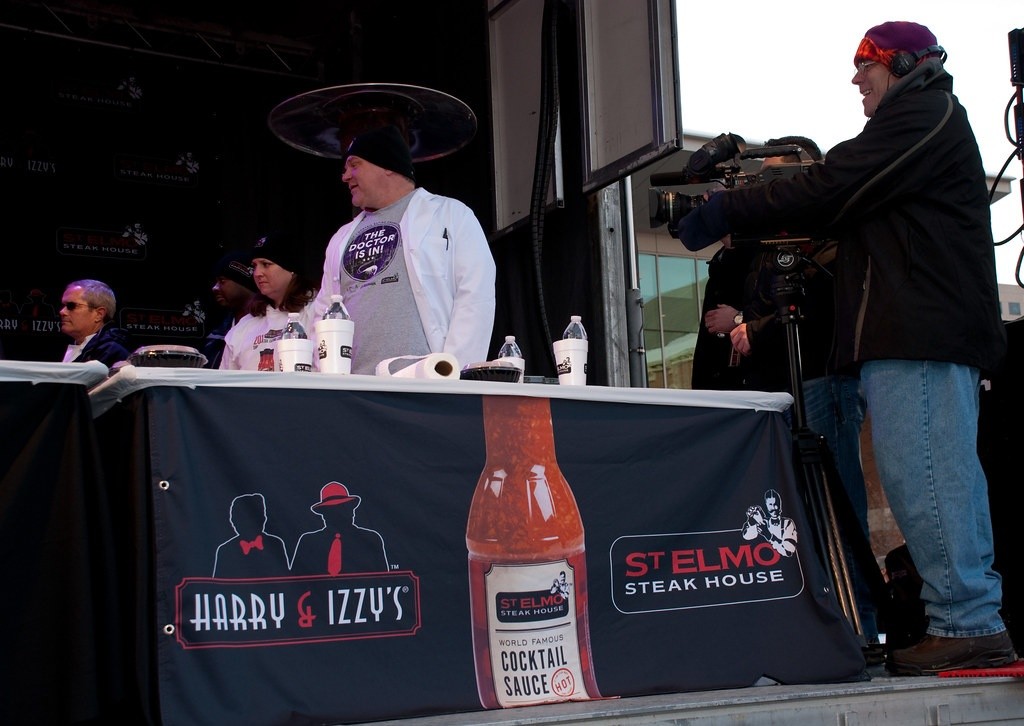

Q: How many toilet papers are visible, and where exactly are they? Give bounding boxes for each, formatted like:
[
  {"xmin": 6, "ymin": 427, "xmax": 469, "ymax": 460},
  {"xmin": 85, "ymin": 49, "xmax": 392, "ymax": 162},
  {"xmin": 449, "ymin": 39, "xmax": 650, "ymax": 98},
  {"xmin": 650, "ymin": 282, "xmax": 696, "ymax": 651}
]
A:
[{"xmin": 374, "ymin": 353, "xmax": 459, "ymax": 382}]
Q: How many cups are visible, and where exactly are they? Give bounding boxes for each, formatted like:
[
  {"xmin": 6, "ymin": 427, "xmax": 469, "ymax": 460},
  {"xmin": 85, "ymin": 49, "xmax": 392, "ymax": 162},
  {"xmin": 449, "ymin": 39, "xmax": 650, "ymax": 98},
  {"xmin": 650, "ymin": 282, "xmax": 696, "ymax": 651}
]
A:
[
  {"xmin": 314, "ymin": 319, "xmax": 356, "ymax": 374},
  {"xmin": 552, "ymin": 338, "xmax": 587, "ymax": 384},
  {"xmin": 277, "ymin": 338, "xmax": 313, "ymax": 372},
  {"xmin": 499, "ymin": 356, "xmax": 525, "ymax": 384}
]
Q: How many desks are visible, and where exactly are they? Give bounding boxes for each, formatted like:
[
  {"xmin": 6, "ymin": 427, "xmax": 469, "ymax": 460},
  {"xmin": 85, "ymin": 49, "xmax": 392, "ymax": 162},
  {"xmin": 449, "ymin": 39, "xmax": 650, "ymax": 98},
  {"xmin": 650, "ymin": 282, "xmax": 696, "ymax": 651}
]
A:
[
  {"xmin": 91, "ymin": 363, "xmax": 866, "ymax": 726},
  {"xmin": 0, "ymin": 359, "xmax": 134, "ymax": 726}
]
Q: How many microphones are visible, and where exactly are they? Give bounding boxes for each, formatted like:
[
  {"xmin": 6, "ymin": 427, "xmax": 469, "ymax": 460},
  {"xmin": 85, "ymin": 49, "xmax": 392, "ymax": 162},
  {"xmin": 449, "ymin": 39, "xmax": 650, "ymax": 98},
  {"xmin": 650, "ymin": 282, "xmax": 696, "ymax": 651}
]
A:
[{"xmin": 650, "ymin": 167, "xmax": 726, "ymax": 186}]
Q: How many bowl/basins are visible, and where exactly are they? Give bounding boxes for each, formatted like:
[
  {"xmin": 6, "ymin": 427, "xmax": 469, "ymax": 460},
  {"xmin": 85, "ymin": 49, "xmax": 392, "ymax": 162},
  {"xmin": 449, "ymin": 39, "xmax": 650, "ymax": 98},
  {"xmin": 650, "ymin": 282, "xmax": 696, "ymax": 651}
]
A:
[{"xmin": 128, "ymin": 345, "xmax": 208, "ymax": 369}]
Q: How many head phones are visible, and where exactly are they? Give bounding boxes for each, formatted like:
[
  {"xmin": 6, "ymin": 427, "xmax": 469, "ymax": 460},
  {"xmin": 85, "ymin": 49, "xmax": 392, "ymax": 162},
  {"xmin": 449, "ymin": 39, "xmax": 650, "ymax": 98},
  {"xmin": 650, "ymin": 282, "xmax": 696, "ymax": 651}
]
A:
[{"xmin": 888, "ymin": 45, "xmax": 948, "ymax": 78}]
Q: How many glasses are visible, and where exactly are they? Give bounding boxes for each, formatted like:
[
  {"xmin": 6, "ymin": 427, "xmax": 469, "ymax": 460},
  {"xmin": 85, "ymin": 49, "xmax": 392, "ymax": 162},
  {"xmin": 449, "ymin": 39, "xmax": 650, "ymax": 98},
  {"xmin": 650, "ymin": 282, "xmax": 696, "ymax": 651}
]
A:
[
  {"xmin": 854, "ymin": 62, "xmax": 878, "ymax": 75},
  {"xmin": 58, "ymin": 302, "xmax": 97, "ymax": 310}
]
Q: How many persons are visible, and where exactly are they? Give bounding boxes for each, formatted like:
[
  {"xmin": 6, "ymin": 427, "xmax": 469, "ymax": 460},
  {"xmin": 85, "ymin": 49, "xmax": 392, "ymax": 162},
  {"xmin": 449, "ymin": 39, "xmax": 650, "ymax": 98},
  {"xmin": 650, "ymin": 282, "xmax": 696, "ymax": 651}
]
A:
[
  {"xmin": 203, "ymin": 240, "xmax": 319, "ymax": 371},
  {"xmin": 691, "ymin": 136, "xmax": 885, "ymax": 666},
  {"xmin": 59, "ymin": 279, "xmax": 133, "ymax": 368},
  {"xmin": 678, "ymin": 21, "xmax": 1018, "ymax": 676},
  {"xmin": 313, "ymin": 126, "xmax": 496, "ymax": 376}
]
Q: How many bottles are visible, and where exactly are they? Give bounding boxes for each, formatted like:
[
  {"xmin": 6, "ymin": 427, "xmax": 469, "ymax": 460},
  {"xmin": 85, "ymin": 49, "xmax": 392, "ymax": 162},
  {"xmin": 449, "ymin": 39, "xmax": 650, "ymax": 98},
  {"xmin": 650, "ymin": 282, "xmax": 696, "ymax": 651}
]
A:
[
  {"xmin": 562, "ymin": 315, "xmax": 587, "ymax": 340},
  {"xmin": 282, "ymin": 312, "xmax": 308, "ymax": 339},
  {"xmin": 322, "ymin": 295, "xmax": 352, "ymax": 320},
  {"xmin": 498, "ymin": 335, "xmax": 522, "ymax": 359},
  {"xmin": 466, "ymin": 395, "xmax": 602, "ymax": 709}
]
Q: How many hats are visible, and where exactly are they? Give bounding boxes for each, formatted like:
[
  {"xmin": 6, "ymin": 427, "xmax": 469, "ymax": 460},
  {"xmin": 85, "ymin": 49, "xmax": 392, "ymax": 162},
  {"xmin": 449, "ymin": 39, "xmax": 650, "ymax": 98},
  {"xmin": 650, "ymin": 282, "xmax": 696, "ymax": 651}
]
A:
[
  {"xmin": 213, "ymin": 253, "xmax": 257, "ymax": 293},
  {"xmin": 854, "ymin": 21, "xmax": 939, "ymax": 80},
  {"xmin": 342, "ymin": 126, "xmax": 416, "ymax": 182},
  {"xmin": 250, "ymin": 232, "xmax": 310, "ymax": 276}
]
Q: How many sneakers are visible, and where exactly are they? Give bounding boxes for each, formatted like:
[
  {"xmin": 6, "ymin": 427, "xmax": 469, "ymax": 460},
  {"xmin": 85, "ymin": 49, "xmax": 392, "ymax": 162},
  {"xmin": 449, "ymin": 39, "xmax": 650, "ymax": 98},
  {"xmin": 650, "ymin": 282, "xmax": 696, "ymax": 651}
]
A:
[{"xmin": 886, "ymin": 629, "xmax": 1019, "ymax": 676}]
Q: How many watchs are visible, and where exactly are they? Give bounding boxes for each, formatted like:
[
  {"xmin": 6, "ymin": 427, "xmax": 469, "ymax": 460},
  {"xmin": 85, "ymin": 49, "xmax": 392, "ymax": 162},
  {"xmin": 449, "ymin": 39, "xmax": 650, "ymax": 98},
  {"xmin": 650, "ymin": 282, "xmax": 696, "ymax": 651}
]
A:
[{"xmin": 733, "ymin": 311, "xmax": 743, "ymax": 326}]
